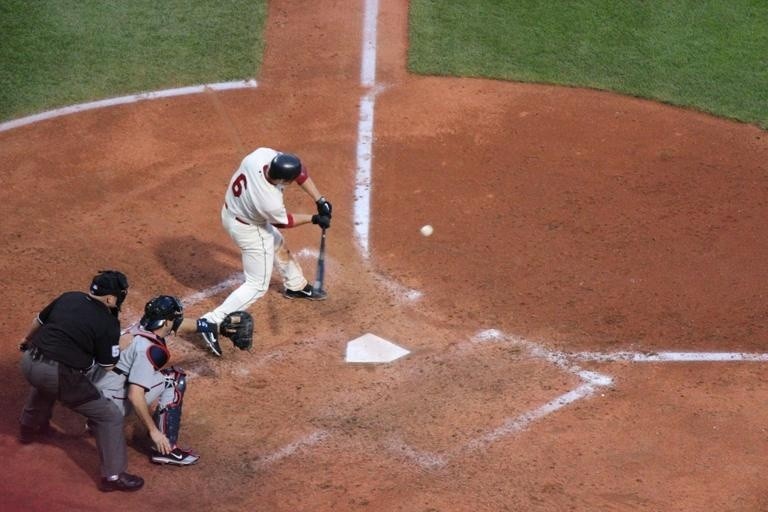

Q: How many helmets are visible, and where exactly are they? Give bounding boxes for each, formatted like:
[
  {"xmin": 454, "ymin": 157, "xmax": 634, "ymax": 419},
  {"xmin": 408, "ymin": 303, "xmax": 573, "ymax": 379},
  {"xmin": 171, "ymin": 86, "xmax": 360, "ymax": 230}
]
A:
[
  {"xmin": 270, "ymin": 153, "xmax": 302, "ymax": 182},
  {"xmin": 89, "ymin": 268, "xmax": 129, "ymax": 312},
  {"xmin": 138, "ymin": 296, "xmax": 184, "ymax": 337}
]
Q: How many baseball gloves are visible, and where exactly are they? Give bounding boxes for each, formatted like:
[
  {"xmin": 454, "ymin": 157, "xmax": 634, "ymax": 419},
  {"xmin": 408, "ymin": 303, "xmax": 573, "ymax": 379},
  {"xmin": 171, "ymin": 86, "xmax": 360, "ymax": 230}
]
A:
[{"xmin": 220, "ymin": 311, "xmax": 254, "ymax": 349}]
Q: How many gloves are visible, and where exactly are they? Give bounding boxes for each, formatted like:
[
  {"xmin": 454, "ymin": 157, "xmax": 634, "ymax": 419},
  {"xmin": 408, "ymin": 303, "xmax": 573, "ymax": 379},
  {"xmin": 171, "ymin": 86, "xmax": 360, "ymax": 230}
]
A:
[{"xmin": 311, "ymin": 196, "xmax": 333, "ymax": 229}]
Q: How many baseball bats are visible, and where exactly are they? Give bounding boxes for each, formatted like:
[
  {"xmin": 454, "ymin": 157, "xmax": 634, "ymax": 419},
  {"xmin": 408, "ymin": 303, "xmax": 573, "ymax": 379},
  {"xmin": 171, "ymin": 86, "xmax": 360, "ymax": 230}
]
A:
[{"xmin": 312, "ymin": 227, "xmax": 326, "ymax": 296}]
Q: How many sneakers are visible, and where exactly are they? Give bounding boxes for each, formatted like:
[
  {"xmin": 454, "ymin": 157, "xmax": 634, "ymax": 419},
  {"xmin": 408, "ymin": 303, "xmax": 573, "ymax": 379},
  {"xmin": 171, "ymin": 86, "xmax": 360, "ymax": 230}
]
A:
[
  {"xmin": 195, "ymin": 316, "xmax": 224, "ymax": 359},
  {"xmin": 283, "ymin": 283, "xmax": 328, "ymax": 302},
  {"xmin": 99, "ymin": 472, "xmax": 146, "ymax": 492},
  {"xmin": 15, "ymin": 422, "xmax": 53, "ymax": 443},
  {"xmin": 149, "ymin": 441, "xmax": 199, "ymax": 467}
]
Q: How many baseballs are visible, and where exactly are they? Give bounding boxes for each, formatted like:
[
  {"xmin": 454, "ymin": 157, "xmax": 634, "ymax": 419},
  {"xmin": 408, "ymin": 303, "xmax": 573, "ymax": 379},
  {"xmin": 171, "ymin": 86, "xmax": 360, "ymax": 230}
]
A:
[{"xmin": 420, "ymin": 225, "xmax": 433, "ymax": 236}]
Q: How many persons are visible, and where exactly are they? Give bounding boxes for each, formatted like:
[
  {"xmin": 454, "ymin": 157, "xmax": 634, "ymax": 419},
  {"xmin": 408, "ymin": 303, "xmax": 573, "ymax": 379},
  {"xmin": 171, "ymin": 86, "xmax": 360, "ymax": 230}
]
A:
[
  {"xmin": 83, "ymin": 294, "xmax": 213, "ymax": 466},
  {"xmin": 17, "ymin": 270, "xmax": 144, "ymax": 492},
  {"xmin": 199, "ymin": 147, "xmax": 332, "ymax": 357}
]
